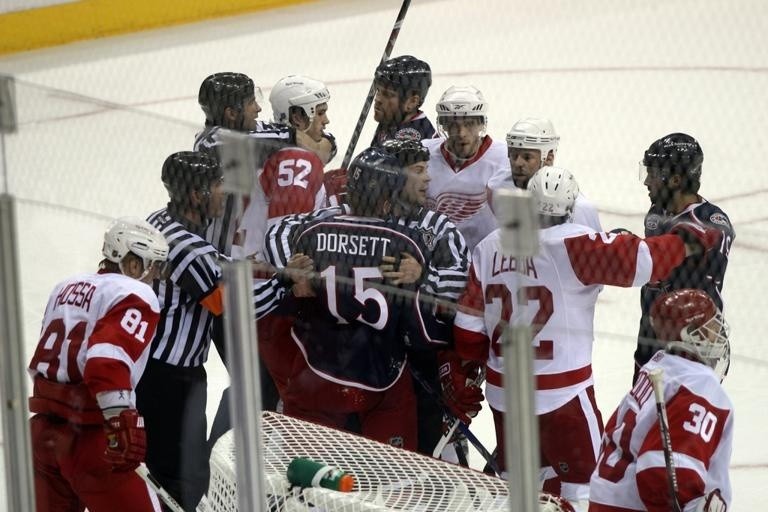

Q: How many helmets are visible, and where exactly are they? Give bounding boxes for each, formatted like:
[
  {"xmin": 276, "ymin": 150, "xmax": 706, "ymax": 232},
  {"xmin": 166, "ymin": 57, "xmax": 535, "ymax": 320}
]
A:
[
  {"xmin": 346, "ymin": 147, "xmax": 408, "ymax": 216},
  {"xmin": 505, "ymin": 118, "xmax": 560, "ymax": 162},
  {"xmin": 101, "ymin": 216, "xmax": 170, "ymax": 265},
  {"xmin": 638, "ymin": 132, "xmax": 704, "ymax": 181},
  {"xmin": 162, "ymin": 151, "xmax": 224, "ymax": 214},
  {"xmin": 269, "ymin": 73, "xmax": 331, "ymax": 123},
  {"xmin": 526, "ymin": 165, "xmax": 579, "ymax": 218},
  {"xmin": 436, "ymin": 84, "xmax": 489, "ymax": 138},
  {"xmin": 374, "ymin": 54, "xmax": 432, "ymax": 93},
  {"xmin": 198, "ymin": 71, "xmax": 254, "ymax": 123},
  {"xmin": 649, "ymin": 288, "xmax": 731, "ymax": 365},
  {"xmin": 379, "ymin": 126, "xmax": 431, "ymax": 167}
]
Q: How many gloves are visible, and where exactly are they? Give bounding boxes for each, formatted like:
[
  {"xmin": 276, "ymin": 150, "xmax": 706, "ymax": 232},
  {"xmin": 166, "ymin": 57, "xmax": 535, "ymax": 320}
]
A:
[
  {"xmin": 670, "ymin": 220, "xmax": 722, "ymax": 256},
  {"xmin": 103, "ymin": 408, "xmax": 147, "ymax": 474},
  {"xmin": 436, "ymin": 348, "xmax": 485, "ymax": 425},
  {"xmin": 324, "ymin": 168, "xmax": 351, "ymax": 205}
]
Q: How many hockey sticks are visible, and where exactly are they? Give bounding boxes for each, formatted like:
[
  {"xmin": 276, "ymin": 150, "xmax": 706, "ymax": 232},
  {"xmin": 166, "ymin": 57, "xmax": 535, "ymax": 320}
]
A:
[
  {"xmin": 379, "ymin": 364, "xmax": 488, "ymax": 492},
  {"xmin": 404, "ymin": 357, "xmax": 562, "ymax": 481}
]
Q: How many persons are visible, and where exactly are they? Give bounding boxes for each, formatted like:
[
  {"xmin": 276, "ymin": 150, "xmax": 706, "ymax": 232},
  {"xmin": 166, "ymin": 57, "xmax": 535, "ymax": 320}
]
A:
[
  {"xmin": 630, "ymin": 131, "xmax": 739, "ymax": 388},
  {"xmin": 588, "ymin": 285, "xmax": 734, "ymax": 511},
  {"xmin": 134, "ymin": 149, "xmax": 316, "ymax": 512},
  {"xmin": 452, "ymin": 161, "xmax": 727, "ymax": 511},
  {"xmin": 189, "ymin": 55, "xmax": 601, "ymax": 511},
  {"xmin": 27, "ymin": 213, "xmax": 174, "ymax": 511}
]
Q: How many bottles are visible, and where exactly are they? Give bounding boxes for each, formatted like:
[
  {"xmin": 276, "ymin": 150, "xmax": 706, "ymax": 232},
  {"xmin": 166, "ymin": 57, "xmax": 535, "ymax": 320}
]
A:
[{"xmin": 284, "ymin": 458, "xmax": 354, "ymax": 492}]
